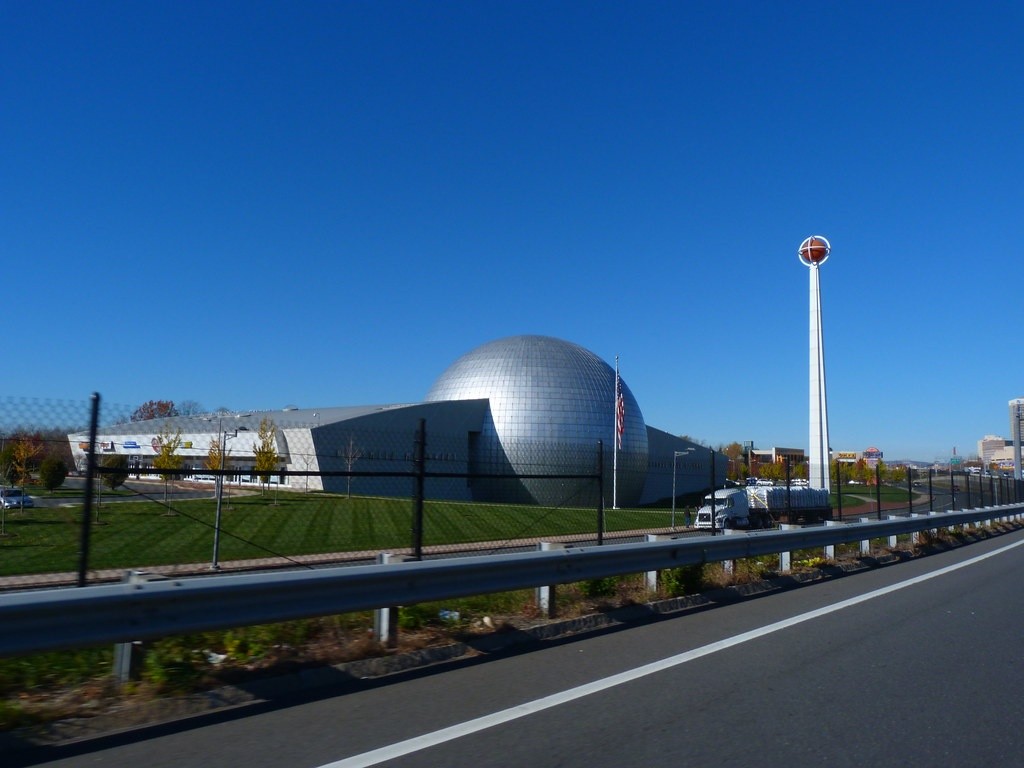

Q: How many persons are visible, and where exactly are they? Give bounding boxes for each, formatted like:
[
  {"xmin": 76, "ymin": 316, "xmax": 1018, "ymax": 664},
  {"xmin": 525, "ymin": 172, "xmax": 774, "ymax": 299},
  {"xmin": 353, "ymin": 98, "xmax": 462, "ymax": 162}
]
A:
[
  {"xmin": 695, "ymin": 505, "xmax": 703, "ymax": 515},
  {"xmin": 683, "ymin": 504, "xmax": 692, "ymax": 528}
]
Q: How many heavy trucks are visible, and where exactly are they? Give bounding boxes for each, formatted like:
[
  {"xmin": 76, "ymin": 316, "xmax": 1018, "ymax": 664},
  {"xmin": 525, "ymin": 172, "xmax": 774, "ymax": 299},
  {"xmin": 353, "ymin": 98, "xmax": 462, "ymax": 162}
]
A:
[{"xmin": 694, "ymin": 485, "xmax": 834, "ymax": 530}]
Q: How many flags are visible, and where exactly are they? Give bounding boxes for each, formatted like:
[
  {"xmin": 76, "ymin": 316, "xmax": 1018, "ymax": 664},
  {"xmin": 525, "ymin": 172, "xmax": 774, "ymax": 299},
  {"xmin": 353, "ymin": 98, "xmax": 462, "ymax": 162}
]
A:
[{"xmin": 615, "ymin": 366, "xmax": 625, "ymax": 449}]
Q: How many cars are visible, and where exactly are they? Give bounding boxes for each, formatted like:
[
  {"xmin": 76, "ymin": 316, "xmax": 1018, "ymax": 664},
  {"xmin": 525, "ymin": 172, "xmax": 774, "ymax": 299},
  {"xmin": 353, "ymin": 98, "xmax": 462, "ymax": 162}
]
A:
[
  {"xmin": 950, "ymin": 485, "xmax": 960, "ymax": 494},
  {"xmin": 848, "ymin": 480, "xmax": 863, "ymax": 485},
  {"xmin": 973, "ymin": 469, "xmax": 1011, "ymax": 477},
  {"xmin": 914, "ymin": 482, "xmax": 922, "ymax": 487},
  {"xmin": 725, "ymin": 477, "xmax": 810, "ymax": 486},
  {"xmin": 0, "ymin": 488, "xmax": 34, "ymax": 510}
]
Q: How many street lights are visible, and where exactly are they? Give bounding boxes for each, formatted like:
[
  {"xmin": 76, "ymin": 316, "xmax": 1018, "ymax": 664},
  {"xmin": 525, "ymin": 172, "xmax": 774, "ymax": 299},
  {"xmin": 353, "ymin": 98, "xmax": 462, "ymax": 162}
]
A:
[
  {"xmin": 210, "ymin": 426, "xmax": 249, "ymax": 571},
  {"xmin": 672, "ymin": 451, "xmax": 690, "ymax": 528}
]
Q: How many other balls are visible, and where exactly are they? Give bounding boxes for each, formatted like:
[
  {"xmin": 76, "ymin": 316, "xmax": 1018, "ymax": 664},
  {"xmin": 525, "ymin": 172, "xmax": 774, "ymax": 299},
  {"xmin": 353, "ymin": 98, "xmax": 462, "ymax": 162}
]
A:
[{"xmin": 804, "ymin": 240, "xmax": 826, "ymax": 261}]
